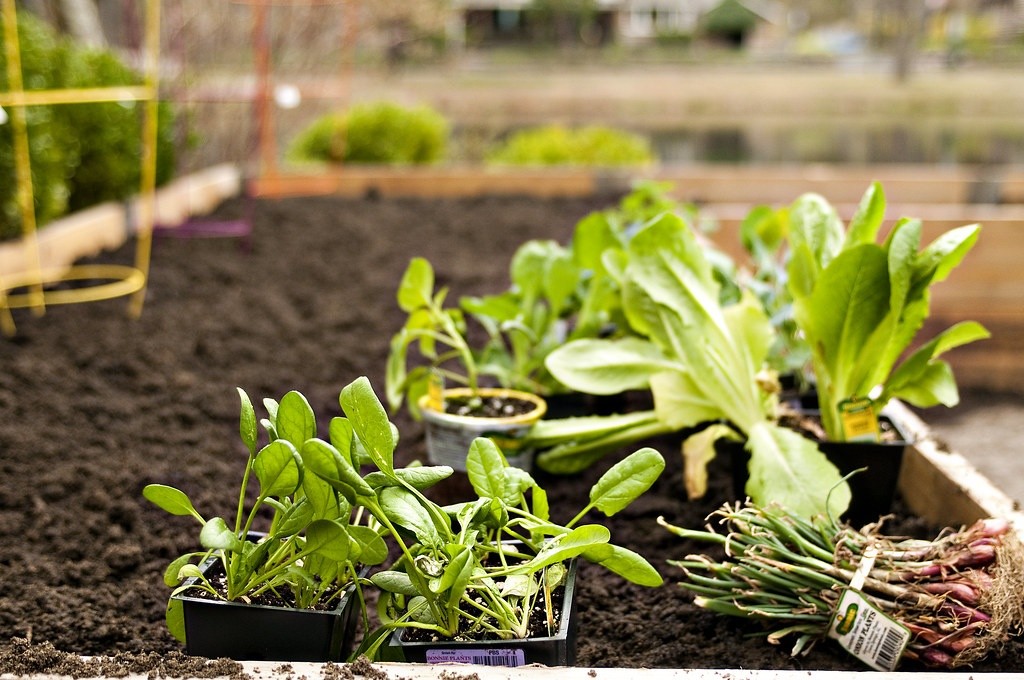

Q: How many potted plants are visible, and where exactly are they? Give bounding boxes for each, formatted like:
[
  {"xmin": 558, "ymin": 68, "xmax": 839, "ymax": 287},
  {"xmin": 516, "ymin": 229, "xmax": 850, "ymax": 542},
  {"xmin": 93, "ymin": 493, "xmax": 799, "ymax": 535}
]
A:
[
  {"xmin": 468, "ymin": 180, "xmax": 994, "ymax": 537},
  {"xmin": 382, "ymin": 256, "xmax": 546, "ymax": 478},
  {"xmin": 297, "ymin": 377, "xmax": 668, "ymax": 674},
  {"xmin": 145, "ymin": 383, "xmax": 456, "ymax": 667}
]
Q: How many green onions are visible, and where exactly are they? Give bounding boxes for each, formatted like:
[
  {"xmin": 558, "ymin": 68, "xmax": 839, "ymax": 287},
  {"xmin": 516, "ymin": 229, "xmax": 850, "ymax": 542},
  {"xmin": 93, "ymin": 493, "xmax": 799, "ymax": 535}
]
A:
[{"xmin": 646, "ymin": 464, "xmax": 1022, "ymax": 672}]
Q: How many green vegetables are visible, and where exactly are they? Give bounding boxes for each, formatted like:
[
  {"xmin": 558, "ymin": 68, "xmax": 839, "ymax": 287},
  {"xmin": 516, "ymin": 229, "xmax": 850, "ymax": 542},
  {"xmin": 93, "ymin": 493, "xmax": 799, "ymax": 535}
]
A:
[
  {"xmin": 120, "ymin": 375, "xmax": 668, "ymax": 634},
  {"xmin": 383, "ymin": 170, "xmax": 988, "ymax": 507}
]
